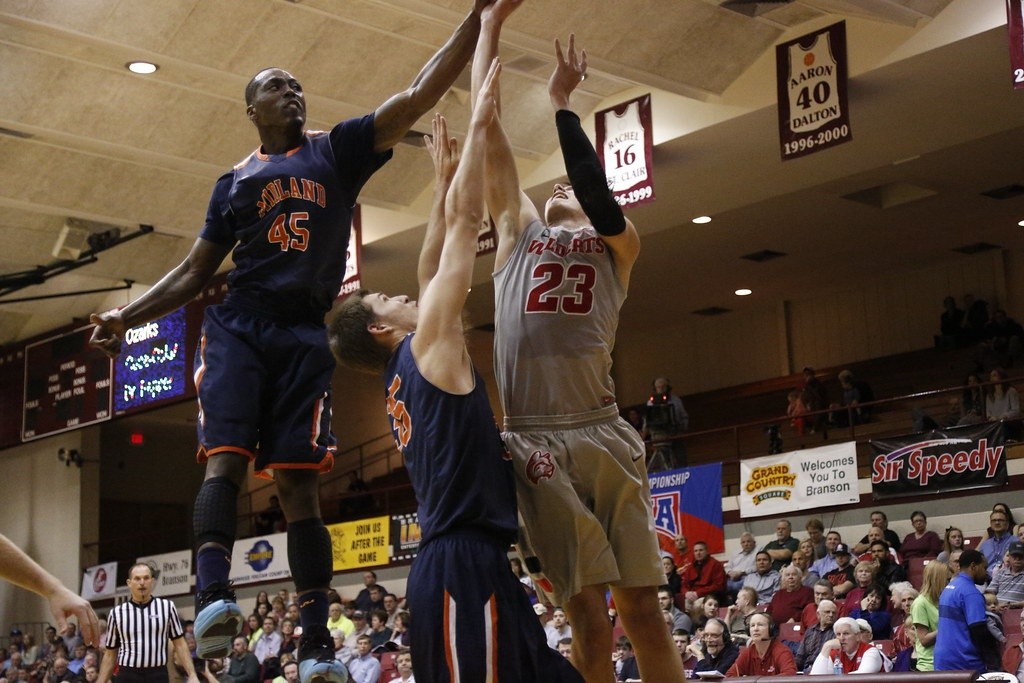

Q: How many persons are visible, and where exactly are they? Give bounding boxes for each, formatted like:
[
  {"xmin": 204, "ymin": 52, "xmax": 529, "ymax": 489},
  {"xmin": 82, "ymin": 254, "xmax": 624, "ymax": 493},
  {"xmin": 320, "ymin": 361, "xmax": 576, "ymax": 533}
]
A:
[
  {"xmin": 725, "ymin": 611, "xmax": 796, "ymax": 678},
  {"xmin": 95, "ymin": 562, "xmax": 199, "ymax": 683},
  {"xmin": 0, "ymin": 294, "xmax": 1024, "ymax": 683},
  {"xmin": 933, "ymin": 549, "xmax": 1005, "ymax": 678},
  {"xmin": 0, "ymin": 533, "xmax": 100, "ymax": 650},
  {"xmin": 809, "ymin": 617, "xmax": 888, "ymax": 675},
  {"xmin": 470, "ymin": 0, "xmax": 686, "ymax": 683},
  {"xmin": 87, "ymin": 0, "xmax": 500, "ymax": 683},
  {"xmin": 327, "ymin": 54, "xmax": 586, "ymax": 683}
]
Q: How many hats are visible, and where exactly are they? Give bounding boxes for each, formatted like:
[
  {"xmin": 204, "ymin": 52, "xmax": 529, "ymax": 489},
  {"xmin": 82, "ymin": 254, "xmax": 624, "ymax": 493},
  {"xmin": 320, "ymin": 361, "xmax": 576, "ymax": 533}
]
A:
[
  {"xmin": 519, "ymin": 577, "xmax": 532, "ymax": 590},
  {"xmin": 353, "ymin": 609, "xmax": 367, "ymax": 618},
  {"xmin": 832, "ymin": 543, "xmax": 852, "ymax": 555},
  {"xmin": 856, "ymin": 619, "xmax": 872, "ymax": 633},
  {"xmin": 1009, "ymin": 541, "xmax": 1024, "ymax": 555},
  {"xmin": 10, "ymin": 629, "xmax": 23, "ymax": 638},
  {"xmin": 292, "ymin": 627, "xmax": 302, "ymax": 637},
  {"xmin": 533, "ymin": 604, "xmax": 547, "ymax": 615}
]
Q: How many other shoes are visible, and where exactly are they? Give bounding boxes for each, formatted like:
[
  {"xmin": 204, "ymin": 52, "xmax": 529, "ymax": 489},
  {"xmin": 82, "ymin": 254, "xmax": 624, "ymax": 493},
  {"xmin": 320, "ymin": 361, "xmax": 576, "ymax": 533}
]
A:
[{"xmin": 608, "ymin": 615, "xmax": 616, "ymax": 627}]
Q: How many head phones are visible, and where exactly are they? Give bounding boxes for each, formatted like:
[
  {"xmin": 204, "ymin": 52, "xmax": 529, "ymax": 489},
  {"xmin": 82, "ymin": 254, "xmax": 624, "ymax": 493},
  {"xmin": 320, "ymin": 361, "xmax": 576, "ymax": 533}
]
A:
[
  {"xmin": 713, "ymin": 617, "xmax": 730, "ymax": 644},
  {"xmin": 744, "ymin": 612, "xmax": 780, "ymax": 637}
]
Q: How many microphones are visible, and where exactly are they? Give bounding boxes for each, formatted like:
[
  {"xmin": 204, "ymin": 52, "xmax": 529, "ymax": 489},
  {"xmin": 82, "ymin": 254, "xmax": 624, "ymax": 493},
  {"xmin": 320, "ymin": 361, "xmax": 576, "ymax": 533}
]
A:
[{"xmin": 761, "ymin": 632, "xmax": 779, "ymax": 641}]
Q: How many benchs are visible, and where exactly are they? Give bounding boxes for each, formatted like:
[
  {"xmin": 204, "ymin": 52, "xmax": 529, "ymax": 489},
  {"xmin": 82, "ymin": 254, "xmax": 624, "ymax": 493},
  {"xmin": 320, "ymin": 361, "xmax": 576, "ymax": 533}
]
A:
[
  {"xmin": 323, "ymin": 463, "xmax": 416, "ymax": 516},
  {"xmin": 618, "ymin": 335, "xmax": 1024, "ymax": 496}
]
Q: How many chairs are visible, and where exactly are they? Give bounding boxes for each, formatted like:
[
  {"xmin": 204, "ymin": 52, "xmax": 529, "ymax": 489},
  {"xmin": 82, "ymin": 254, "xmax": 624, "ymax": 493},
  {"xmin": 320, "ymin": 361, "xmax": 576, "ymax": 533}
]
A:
[
  {"xmin": 963, "ymin": 536, "xmax": 982, "ymax": 550},
  {"xmin": 379, "ymin": 651, "xmax": 400, "ymax": 682},
  {"xmin": 874, "ymin": 640, "xmax": 894, "ymax": 656},
  {"xmin": 908, "ymin": 557, "xmax": 937, "ymax": 593},
  {"xmin": 779, "ymin": 623, "xmax": 802, "ymax": 643}
]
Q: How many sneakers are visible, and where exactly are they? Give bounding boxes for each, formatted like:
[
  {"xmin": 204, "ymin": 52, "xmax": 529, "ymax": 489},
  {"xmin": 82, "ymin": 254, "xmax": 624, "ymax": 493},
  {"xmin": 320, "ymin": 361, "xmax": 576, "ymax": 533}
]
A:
[
  {"xmin": 297, "ymin": 624, "xmax": 348, "ymax": 683},
  {"xmin": 193, "ymin": 581, "xmax": 243, "ymax": 659}
]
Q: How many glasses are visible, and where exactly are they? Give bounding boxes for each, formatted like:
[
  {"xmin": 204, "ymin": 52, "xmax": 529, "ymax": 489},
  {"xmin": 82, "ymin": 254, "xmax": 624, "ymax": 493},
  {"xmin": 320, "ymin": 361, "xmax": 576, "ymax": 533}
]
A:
[
  {"xmin": 991, "ymin": 519, "xmax": 1008, "ymax": 523},
  {"xmin": 703, "ymin": 632, "xmax": 723, "ymax": 639}
]
